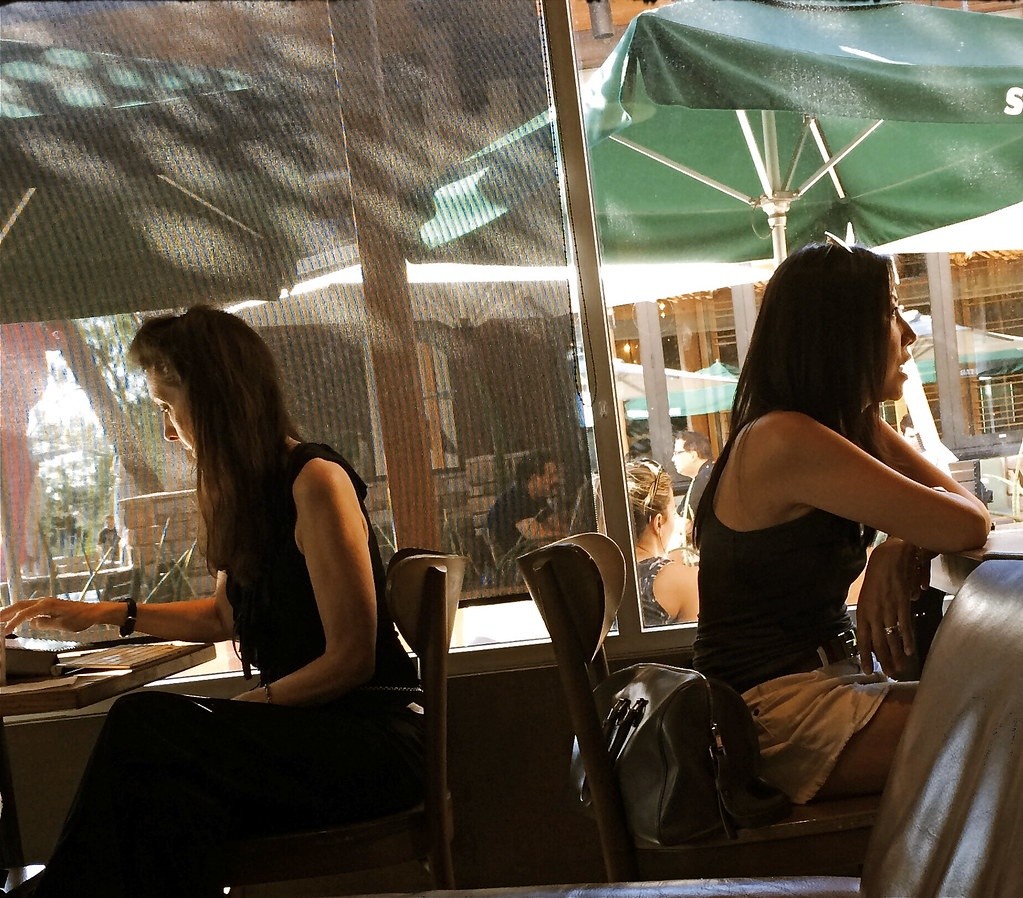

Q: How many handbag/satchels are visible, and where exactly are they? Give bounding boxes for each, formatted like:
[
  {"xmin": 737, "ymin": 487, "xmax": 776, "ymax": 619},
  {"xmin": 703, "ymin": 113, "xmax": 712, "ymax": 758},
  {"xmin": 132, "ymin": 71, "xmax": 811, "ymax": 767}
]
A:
[{"xmin": 570, "ymin": 659, "xmax": 791, "ymax": 847}]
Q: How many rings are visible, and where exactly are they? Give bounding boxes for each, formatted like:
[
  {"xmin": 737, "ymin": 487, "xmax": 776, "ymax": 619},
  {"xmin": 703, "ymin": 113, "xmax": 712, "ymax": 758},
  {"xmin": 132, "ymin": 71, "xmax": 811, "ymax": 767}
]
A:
[{"xmin": 884, "ymin": 625, "xmax": 898, "ymax": 635}]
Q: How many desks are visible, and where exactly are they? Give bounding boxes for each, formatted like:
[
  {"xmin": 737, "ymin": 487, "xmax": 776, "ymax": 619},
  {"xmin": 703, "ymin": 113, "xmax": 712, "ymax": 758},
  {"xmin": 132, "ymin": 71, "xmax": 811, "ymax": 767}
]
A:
[
  {"xmin": 919, "ymin": 523, "xmax": 1023, "ymax": 660},
  {"xmin": 2, "ymin": 642, "xmax": 218, "ymax": 896}
]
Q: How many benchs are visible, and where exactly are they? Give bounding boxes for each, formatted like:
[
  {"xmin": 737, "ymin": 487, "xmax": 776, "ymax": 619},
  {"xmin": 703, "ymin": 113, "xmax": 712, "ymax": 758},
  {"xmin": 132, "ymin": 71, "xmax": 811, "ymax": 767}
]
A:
[
  {"xmin": 949, "ymin": 460, "xmax": 982, "ymax": 497},
  {"xmin": 145, "ymin": 560, "xmax": 183, "ymax": 604},
  {"xmin": 222, "ymin": 545, "xmax": 468, "ymax": 896}
]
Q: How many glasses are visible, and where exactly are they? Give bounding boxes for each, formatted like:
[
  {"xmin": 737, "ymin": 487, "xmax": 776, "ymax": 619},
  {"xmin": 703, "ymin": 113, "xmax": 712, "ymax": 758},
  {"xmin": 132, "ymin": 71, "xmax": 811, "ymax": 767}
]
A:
[
  {"xmin": 672, "ymin": 451, "xmax": 689, "ymax": 456},
  {"xmin": 639, "ymin": 458, "xmax": 666, "ymax": 524},
  {"xmin": 823, "ymin": 230, "xmax": 853, "ymax": 259}
]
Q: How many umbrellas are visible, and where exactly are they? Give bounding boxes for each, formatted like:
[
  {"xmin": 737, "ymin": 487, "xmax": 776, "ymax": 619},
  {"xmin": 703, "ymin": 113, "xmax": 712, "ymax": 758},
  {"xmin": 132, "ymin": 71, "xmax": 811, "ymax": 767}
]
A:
[
  {"xmin": 616, "ymin": 360, "xmax": 745, "ymax": 457},
  {"xmin": 407, "ymin": 0, "xmax": 1023, "ymax": 276},
  {"xmin": 887, "ymin": 309, "xmax": 1023, "ymax": 393},
  {"xmin": 570, "ymin": 346, "xmax": 739, "ymax": 403},
  {"xmin": 0, "ymin": 41, "xmax": 311, "ymax": 327}
]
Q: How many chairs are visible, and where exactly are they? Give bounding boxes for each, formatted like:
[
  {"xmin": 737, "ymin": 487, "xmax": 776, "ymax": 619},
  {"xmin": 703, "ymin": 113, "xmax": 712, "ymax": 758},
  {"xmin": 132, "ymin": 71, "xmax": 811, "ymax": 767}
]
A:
[
  {"xmin": 517, "ymin": 531, "xmax": 879, "ymax": 881},
  {"xmin": 456, "ymin": 519, "xmax": 519, "ymax": 590},
  {"xmin": 101, "ymin": 567, "xmax": 141, "ymax": 604}
]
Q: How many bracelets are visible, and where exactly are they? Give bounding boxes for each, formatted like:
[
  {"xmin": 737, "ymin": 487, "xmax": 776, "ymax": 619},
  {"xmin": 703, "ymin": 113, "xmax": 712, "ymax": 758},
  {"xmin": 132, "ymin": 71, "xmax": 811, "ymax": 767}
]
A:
[
  {"xmin": 118, "ymin": 598, "xmax": 138, "ymax": 637},
  {"xmin": 261, "ymin": 683, "xmax": 276, "ymax": 706},
  {"xmin": 931, "ymin": 485, "xmax": 947, "ymax": 492}
]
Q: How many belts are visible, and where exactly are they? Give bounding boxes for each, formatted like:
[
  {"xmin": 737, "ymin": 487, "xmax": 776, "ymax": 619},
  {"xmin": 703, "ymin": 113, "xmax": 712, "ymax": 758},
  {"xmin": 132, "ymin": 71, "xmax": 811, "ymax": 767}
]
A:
[{"xmin": 737, "ymin": 624, "xmax": 861, "ymax": 694}]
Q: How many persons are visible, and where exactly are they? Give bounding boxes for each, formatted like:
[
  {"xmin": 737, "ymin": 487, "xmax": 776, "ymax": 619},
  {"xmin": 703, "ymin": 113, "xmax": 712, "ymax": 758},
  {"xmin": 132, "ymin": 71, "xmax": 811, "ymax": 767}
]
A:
[
  {"xmin": 672, "ymin": 430, "xmax": 718, "ymax": 517},
  {"xmin": 897, "ymin": 412, "xmax": 963, "ymax": 464},
  {"xmin": 572, "ymin": 458, "xmax": 702, "ymax": 634},
  {"xmin": 484, "ymin": 451, "xmax": 571, "ymax": 554},
  {"xmin": 0, "ymin": 301, "xmax": 405, "ymax": 897},
  {"xmin": 95, "ymin": 514, "xmax": 120, "ymax": 561},
  {"xmin": 684, "ymin": 237, "xmax": 996, "ymax": 805}
]
